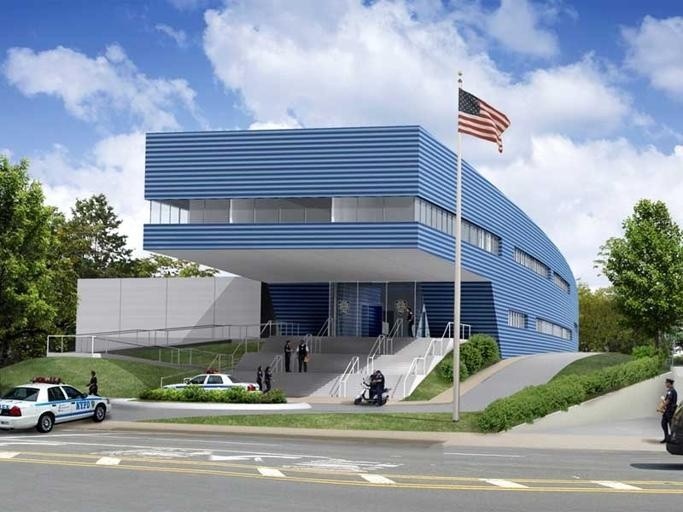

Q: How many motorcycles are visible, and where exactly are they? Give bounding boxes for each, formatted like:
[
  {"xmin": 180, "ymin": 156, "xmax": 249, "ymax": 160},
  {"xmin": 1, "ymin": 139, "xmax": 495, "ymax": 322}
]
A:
[{"xmin": 354, "ymin": 378, "xmax": 391, "ymax": 407}]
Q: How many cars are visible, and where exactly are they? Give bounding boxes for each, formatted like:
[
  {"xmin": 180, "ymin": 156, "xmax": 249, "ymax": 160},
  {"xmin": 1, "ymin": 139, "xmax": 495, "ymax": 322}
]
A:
[
  {"xmin": 163, "ymin": 369, "xmax": 259, "ymax": 393},
  {"xmin": 666, "ymin": 400, "xmax": 682, "ymax": 456},
  {"xmin": 0, "ymin": 376, "xmax": 111, "ymax": 434},
  {"xmin": 672, "ymin": 345, "xmax": 682, "ymax": 358}
]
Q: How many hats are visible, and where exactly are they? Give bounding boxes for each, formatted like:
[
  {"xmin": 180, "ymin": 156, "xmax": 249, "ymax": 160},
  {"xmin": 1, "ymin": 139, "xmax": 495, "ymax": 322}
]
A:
[{"xmin": 665, "ymin": 379, "xmax": 674, "ymax": 384}]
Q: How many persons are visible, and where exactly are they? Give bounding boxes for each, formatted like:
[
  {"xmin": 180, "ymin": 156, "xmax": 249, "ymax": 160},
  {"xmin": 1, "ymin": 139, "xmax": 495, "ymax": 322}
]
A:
[
  {"xmin": 85, "ymin": 370, "xmax": 100, "ymax": 396},
  {"xmin": 362, "ymin": 369, "xmax": 384, "ymax": 406},
  {"xmin": 256, "ymin": 365, "xmax": 264, "ymax": 391},
  {"xmin": 583, "ymin": 344, "xmax": 589, "ymax": 352},
  {"xmin": 660, "ymin": 378, "xmax": 678, "ymax": 444},
  {"xmin": 295, "ymin": 339, "xmax": 310, "ymax": 373},
  {"xmin": 283, "ymin": 339, "xmax": 294, "ymax": 373},
  {"xmin": 263, "ymin": 366, "xmax": 273, "ymax": 394},
  {"xmin": 406, "ymin": 306, "xmax": 414, "ymax": 337}
]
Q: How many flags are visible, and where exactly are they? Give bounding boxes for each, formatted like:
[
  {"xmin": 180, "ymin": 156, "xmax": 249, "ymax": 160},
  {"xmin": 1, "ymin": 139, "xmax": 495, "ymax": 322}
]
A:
[{"xmin": 457, "ymin": 86, "xmax": 511, "ymax": 154}]
